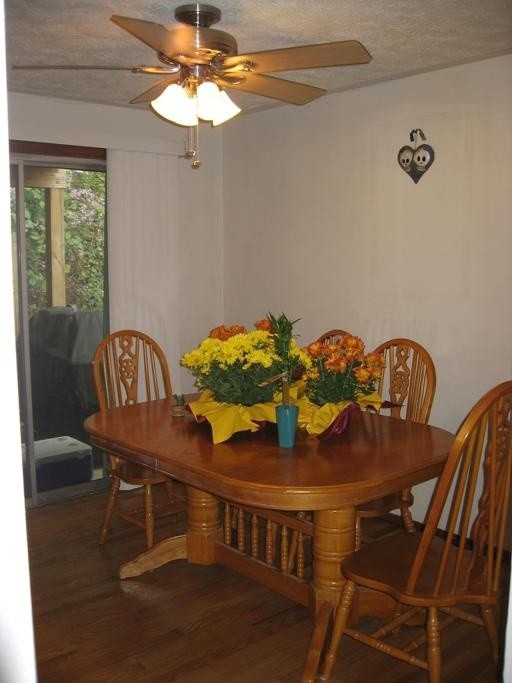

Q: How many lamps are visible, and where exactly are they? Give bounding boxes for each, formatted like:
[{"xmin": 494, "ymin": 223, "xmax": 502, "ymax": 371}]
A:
[{"xmin": 150, "ymin": 75, "xmax": 242, "ymax": 127}]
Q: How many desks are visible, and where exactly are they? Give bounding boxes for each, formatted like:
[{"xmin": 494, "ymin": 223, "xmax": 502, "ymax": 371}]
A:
[{"xmin": 82, "ymin": 394, "xmax": 457, "ymax": 683}]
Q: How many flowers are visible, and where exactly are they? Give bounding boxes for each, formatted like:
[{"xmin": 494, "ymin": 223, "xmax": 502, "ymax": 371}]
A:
[{"xmin": 181, "ymin": 310, "xmax": 389, "ymax": 405}]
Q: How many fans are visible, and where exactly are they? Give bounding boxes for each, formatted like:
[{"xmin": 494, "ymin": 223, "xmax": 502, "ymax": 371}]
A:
[{"xmin": 12, "ymin": 2, "xmax": 372, "ymax": 106}]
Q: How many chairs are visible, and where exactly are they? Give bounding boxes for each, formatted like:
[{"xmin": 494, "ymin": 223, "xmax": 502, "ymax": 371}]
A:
[
  {"xmin": 288, "ymin": 339, "xmax": 438, "ymax": 573},
  {"xmin": 319, "ymin": 381, "xmax": 511, "ymax": 681},
  {"xmin": 93, "ymin": 328, "xmax": 193, "ymax": 549},
  {"xmin": 224, "ymin": 334, "xmax": 362, "ymax": 557}
]
